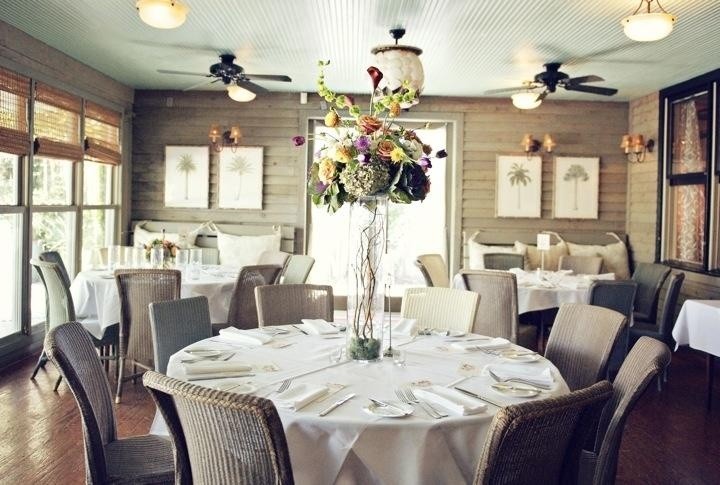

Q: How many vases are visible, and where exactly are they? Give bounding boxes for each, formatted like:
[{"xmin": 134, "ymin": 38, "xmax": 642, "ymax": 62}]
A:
[{"xmin": 345, "ymin": 195, "xmax": 387, "ymax": 358}]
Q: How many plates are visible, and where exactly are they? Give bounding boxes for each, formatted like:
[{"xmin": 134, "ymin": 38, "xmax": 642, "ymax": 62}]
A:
[
  {"xmin": 434, "ymin": 330, "xmax": 465, "ymax": 337},
  {"xmin": 184, "ymin": 348, "xmax": 222, "ymax": 358},
  {"xmin": 260, "ymin": 327, "xmax": 288, "ymax": 334},
  {"xmin": 368, "ymin": 399, "xmax": 415, "ymax": 417},
  {"xmin": 490, "ymin": 382, "xmax": 538, "ymax": 397},
  {"xmin": 500, "ymin": 351, "xmax": 540, "ymax": 363}
]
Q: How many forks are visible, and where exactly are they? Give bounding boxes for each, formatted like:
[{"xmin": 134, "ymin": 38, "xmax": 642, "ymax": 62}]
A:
[
  {"xmin": 475, "ymin": 344, "xmax": 540, "ymax": 356},
  {"xmin": 426, "ymin": 327, "xmax": 434, "ymax": 336},
  {"xmin": 395, "ymin": 388, "xmax": 441, "ymax": 420},
  {"xmin": 418, "ymin": 327, "xmax": 428, "ymax": 335},
  {"xmin": 265, "ymin": 376, "xmax": 292, "ymax": 397},
  {"xmin": 487, "ymin": 368, "xmax": 552, "ymax": 390},
  {"xmin": 405, "ymin": 388, "xmax": 448, "ymax": 417}
]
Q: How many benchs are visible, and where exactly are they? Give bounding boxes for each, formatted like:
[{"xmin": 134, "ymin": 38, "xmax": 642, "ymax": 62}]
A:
[
  {"xmin": 462, "ymin": 227, "xmax": 629, "ymax": 272},
  {"xmin": 124, "ymin": 219, "xmax": 296, "ymax": 263}
]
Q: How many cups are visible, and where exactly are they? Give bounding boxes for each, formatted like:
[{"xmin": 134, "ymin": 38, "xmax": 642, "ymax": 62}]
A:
[
  {"xmin": 132, "ymin": 247, "xmax": 146, "ymax": 269},
  {"xmin": 391, "ymin": 348, "xmax": 407, "ymax": 367},
  {"xmin": 191, "ymin": 248, "xmax": 202, "ymax": 280},
  {"xmin": 151, "ymin": 248, "xmax": 163, "ymax": 270},
  {"xmin": 177, "ymin": 249, "xmax": 189, "ymax": 279},
  {"xmin": 124, "ymin": 246, "xmax": 133, "ymax": 270},
  {"xmin": 327, "ymin": 346, "xmax": 342, "ymax": 368},
  {"xmin": 108, "ymin": 245, "xmax": 120, "ymax": 274}
]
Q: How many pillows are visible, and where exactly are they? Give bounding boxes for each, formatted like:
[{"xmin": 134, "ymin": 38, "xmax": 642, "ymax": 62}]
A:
[
  {"xmin": 566, "ymin": 231, "xmax": 630, "ymax": 281},
  {"xmin": 208, "ymin": 223, "xmax": 282, "ymax": 267},
  {"xmin": 467, "ymin": 230, "xmax": 527, "ymax": 271},
  {"xmin": 133, "ymin": 220, "xmax": 208, "ymax": 250},
  {"xmin": 514, "ymin": 231, "xmax": 569, "ymax": 272}
]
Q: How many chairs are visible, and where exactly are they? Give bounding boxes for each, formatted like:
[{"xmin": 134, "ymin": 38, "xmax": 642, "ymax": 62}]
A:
[
  {"xmin": 471, "ymin": 380, "xmax": 613, "ymax": 484},
  {"xmin": 147, "ymin": 296, "xmax": 215, "ymax": 372},
  {"xmin": 557, "ymin": 256, "xmax": 604, "ymax": 275},
  {"xmin": 400, "ymin": 286, "xmax": 478, "ymax": 337},
  {"xmin": 543, "ymin": 303, "xmax": 620, "ymax": 389},
  {"xmin": 458, "ymin": 269, "xmax": 519, "ymax": 343},
  {"xmin": 40, "ymin": 250, "xmax": 71, "ymax": 289},
  {"xmin": 183, "ymin": 248, "xmax": 218, "ymax": 265},
  {"xmin": 29, "ymin": 258, "xmax": 120, "ymax": 390},
  {"xmin": 586, "ymin": 279, "xmax": 637, "ymax": 371},
  {"xmin": 110, "ymin": 268, "xmax": 181, "ymax": 404},
  {"xmin": 579, "ymin": 335, "xmax": 672, "ymax": 484},
  {"xmin": 43, "ymin": 320, "xmax": 178, "ymax": 483},
  {"xmin": 253, "ymin": 283, "xmax": 334, "ymax": 330},
  {"xmin": 276, "ymin": 255, "xmax": 314, "ymax": 284},
  {"xmin": 481, "ymin": 253, "xmax": 524, "ymax": 271},
  {"xmin": 415, "ymin": 254, "xmax": 450, "ymax": 288},
  {"xmin": 630, "ymin": 269, "xmax": 685, "ymax": 391},
  {"xmin": 629, "ymin": 262, "xmax": 671, "ymax": 321},
  {"xmin": 138, "ymin": 370, "xmax": 296, "ymax": 485},
  {"xmin": 256, "ymin": 249, "xmax": 289, "ymax": 269},
  {"xmin": 212, "ymin": 264, "xmax": 282, "ymax": 334}
]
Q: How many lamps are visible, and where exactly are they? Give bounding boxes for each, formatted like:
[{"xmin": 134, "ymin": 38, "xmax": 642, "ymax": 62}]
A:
[
  {"xmin": 371, "ymin": 29, "xmax": 424, "ymax": 110},
  {"xmin": 620, "ymin": 134, "xmax": 634, "ymax": 163},
  {"xmin": 633, "ymin": 133, "xmax": 654, "ymax": 163},
  {"xmin": 208, "ymin": 122, "xmax": 223, "ymax": 153},
  {"xmin": 226, "ymin": 83, "xmax": 256, "ymax": 103},
  {"xmin": 228, "ymin": 124, "xmax": 243, "ymax": 147},
  {"xmin": 620, "ymin": 0, "xmax": 677, "ymax": 43},
  {"xmin": 542, "ymin": 133, "xmax": 556, "ymax": 153},
  {"xmin": 520, "ymin": 131, "xmax": 537, "ymax": 160},
  {"xmin": 510, "ymin": 82, "xmax": 543, "ymax": 110}
]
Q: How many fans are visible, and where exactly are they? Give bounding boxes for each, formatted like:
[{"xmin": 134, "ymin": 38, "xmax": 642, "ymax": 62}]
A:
[
  {"xmin": 182, "ymin": 54, "xmax": 292, "ymax": 99},
  {"xmin": 531, "ymin": 63, "xmax": 618, "ymax": 101}
]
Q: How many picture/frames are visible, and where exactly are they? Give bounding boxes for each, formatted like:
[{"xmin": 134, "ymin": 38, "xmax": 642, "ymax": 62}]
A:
[
  {"xmin": 495, "ymin": 153, "xmax": 543, "ymax": 219},
  {"xmin": 551, "ymin": 154, "xmax": 600, "ymax": 220},
  {"xmin": 164, "ymin": 144, "xmax": 210, "ymax": 209},
  {"xmin": 216, "ymin": 145, "xmax": 263, "ymax": 209}
]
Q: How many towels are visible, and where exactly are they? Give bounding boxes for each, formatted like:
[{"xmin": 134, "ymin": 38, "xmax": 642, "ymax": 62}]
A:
[
  {"xmin": 510, "ymin": 267, "xmax": 533, "ymax": 281},
  {"xmin": 452, "ymin": 335, "xmax": 512, "ymax": 352},
  {"xmin": 387, "ymin": 318, "xmax": 418, "ymax": 338},
  {"xmin": 272, "ymin": 380, "xmax": 329, "ymax": 413},
  {"xmin": 219, "ymin": 326, "xmax": 273, "ymax": 349},
  {"xmin": 301, "ymin": 318, "xmax": 340, "ymax": 339},
  {"xmin": 181, "ymin": 358, "xmax": 256, "ymax": 382},
  {"xmin": 418, "ymin": 384, "xmax": 489, "ymax": 417},
  {"xmin": 483, "ymin": 358, "xmax": 555, "ymax": 388}
]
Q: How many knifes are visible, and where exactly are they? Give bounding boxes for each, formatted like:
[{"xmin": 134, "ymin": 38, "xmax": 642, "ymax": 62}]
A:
[
  {"xmin": 453, "ymin": 386, "xmax": 508, "ymax": 411},
  {"xmin": 318, "ymin": 392, "xmax": 355, "ymax": 416},
  {"xmin": 292, "ymin": 325, "xmax": 308, "ymax": 336},
  {"xmin": 443, "ymin": 338, "xmax": 490, "ymax": 342}
]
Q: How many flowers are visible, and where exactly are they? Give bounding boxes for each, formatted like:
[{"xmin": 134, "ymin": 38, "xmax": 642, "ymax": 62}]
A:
[
  {"xmin": 142, "ymin": 229, "xmax": 180, "ymax": 270},
  {"xmin": 289, "ymin": 58, "xmax": 447, "ymax": 215}
]
